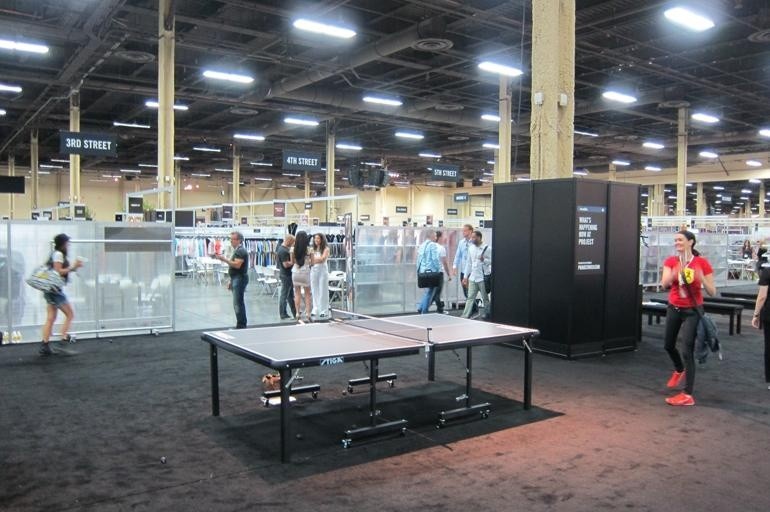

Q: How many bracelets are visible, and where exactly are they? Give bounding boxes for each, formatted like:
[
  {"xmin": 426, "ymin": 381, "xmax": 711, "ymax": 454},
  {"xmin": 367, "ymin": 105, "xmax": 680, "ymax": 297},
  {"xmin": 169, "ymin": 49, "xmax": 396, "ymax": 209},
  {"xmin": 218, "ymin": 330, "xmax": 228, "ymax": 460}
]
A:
[{"xmin": 753, "ymin": 312, "xmax": 760, "ymax": 317}]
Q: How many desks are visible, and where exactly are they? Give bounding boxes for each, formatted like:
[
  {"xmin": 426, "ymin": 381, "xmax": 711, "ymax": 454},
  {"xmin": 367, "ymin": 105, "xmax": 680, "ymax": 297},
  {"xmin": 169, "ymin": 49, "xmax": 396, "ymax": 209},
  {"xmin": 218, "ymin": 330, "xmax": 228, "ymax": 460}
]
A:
[
  {"xmin": 201, "ymin": 311, "xmax": 540, "ymax": 464},
  {"xmin": 87, "ymin": 278, "xmax": 131, "ymax": 315}
]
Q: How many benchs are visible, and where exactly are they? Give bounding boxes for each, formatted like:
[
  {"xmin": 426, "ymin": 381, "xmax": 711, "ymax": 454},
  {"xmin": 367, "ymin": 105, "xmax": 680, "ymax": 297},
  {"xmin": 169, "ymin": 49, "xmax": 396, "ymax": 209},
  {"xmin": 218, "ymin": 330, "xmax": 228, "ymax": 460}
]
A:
[{"xmin": 641, "ymin": 292, "xmax": 759, "ymax": 336}]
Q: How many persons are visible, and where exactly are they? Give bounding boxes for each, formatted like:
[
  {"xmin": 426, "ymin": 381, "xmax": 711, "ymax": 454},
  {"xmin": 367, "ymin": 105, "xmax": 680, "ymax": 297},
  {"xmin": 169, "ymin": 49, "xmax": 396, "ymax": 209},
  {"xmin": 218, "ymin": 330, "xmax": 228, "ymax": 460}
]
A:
[
  {"xmin": 460, "ymin": 231, "xmax": 492, "ymax": 319},
  {"xmin": 216, "ymin": 231, "xmax": 249, "ymax": 329},
  {"xmin": 430, "ymin": 231, "xmax": 451, "ymax": 314},
  {"xmin": 751, "ymin": 267, "xmax": 770, "ymax": 390},
  {"xmin": 740, "ymin": 237, "xmax": 767, "ymax": 276},
  {"xmin": 417, "ymin": 231, "xmax": 441, "ymax": 314},
  {"xmin": 277, "ymin": 234, "xmax": 298, "ymax": 320},
  {"xmin": 658, "ymin": 230, "xmax": 716, "ymax": 406},
  {"xmin": 290, "ymin": 231, "xmax": 314, "ymax": 322},
  {"xmin": 310, "ymin": 233, "xmax": 331, "ymax": 317},
  {"xmin": 453, "ymin": 224, "xmax": 475, "ymax": 313},
  {"xmin": 39, "ymin": 234, "xmax": 83, "ymax": 357}
]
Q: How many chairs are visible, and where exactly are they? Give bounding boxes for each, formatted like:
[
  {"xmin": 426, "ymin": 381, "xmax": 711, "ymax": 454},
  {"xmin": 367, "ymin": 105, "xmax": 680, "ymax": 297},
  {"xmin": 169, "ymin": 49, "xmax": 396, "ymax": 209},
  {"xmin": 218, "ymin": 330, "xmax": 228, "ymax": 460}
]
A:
[
  {"xmin": 131, "ymin": 275, "xmax": 171, "ymax": 317},
  {"xmin": 184, "ymin": 254, "xmax": 354, "ymax": 313}
]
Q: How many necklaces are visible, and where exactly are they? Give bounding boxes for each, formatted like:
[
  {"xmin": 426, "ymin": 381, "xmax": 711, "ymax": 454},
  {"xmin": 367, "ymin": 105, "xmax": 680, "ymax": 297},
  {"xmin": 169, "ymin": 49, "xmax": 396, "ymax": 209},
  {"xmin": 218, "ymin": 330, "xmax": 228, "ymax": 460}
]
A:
[
  {"xmin": 338, "ymin": 235, "xmax": 343, "ymax": 242},
  {"xmin": 290, "ymin": 225, "xmax": 295, "ymax": 235},
  {"xmin": 328, "ymin": 236, "xmax": 333, "ymax": 241}
]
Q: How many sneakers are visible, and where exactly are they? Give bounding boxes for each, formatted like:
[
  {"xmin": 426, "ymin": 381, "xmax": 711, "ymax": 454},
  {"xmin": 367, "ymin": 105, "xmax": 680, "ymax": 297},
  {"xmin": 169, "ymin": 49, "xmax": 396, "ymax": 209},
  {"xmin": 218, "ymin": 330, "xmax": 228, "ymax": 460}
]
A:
[
  {"xmin": 667, "ymin": 369, "xmax": 686, "ymax": 388},
  {"xmin": 665, "ymin": 393, "xmax": 695, "ymax": 405}
]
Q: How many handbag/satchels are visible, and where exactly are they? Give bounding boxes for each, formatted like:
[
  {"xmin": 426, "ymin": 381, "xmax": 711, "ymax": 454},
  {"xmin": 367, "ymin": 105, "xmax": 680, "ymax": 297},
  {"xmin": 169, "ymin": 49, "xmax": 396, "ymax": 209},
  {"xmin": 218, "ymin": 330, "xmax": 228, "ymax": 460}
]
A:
[
  {"xmin": 696, "ymin": 316, "xmax": 720, "ymax": 360},
  {"xmin": 26, "ymin": 265, "xmax": 64, "ymax": 295},
  {"xmin": 484, "ymin": 274, "xmax": 492, "ymax": 293},
  {"xmin": 416, "ymin": 270, "xmax": 440, "ymax": 287}
]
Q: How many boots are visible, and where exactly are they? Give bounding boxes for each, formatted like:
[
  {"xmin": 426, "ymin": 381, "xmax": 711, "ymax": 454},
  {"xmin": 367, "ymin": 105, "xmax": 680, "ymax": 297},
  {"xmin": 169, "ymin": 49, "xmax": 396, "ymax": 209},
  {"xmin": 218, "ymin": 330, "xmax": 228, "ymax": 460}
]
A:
[
  {"xmin": 59, "ymin": 339, "xmax": 77, "ymax": 355},
  {"xmin": 40, "ymin": 340, "xmax": 59, "ymax": 355}
]
